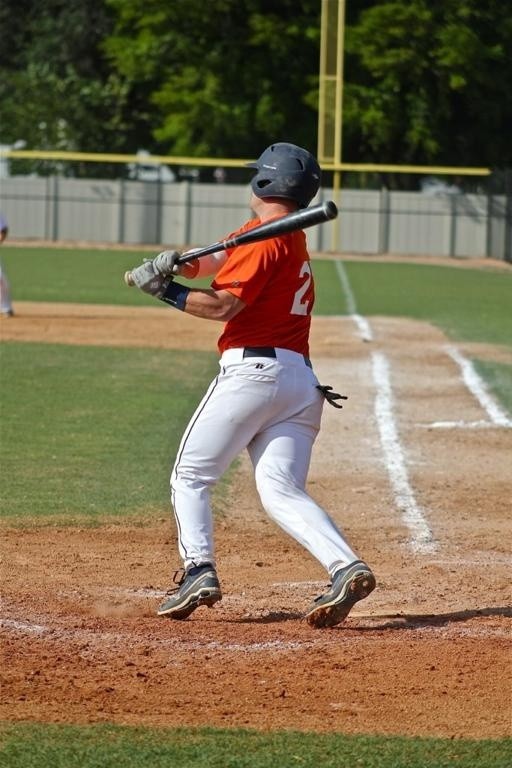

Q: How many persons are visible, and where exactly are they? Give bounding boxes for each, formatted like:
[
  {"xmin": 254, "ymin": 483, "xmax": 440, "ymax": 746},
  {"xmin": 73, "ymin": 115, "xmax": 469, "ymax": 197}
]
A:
[
  {"xmin": 0, "ymin": 208, "xmax": 15, "ymax": 317},
  {"xmin": 130, "ymin": 143, "xmax": 377, "ymax": 627}
]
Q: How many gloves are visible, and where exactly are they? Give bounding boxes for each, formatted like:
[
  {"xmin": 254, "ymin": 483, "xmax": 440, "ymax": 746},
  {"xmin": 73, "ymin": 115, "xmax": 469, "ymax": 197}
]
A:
[
  {"xmin": 129, "ymin": 251, "xmax": 181, "ymax": 301},
  {"xmin": 316, "ymin": 384, "xmax": 348, "ymax": 409}
]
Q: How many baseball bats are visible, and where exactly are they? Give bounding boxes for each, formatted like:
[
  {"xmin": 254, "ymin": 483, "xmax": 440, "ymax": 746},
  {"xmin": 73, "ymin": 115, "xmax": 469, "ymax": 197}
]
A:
[{"xmin": 171, "ymin": 203, "xmax": 338, "ymax": 265}]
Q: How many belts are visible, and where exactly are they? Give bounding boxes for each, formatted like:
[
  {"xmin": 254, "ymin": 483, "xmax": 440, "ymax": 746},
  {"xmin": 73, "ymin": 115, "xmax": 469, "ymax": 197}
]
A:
[{"xmin": 242, "ymin": 347, "xmax": 313, "ymax": 368}]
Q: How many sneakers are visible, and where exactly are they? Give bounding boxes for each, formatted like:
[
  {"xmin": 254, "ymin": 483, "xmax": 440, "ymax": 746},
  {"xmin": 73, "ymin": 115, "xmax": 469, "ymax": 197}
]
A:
[
  {"xmin": 157, "ymin": 567, "xmax": 222, "ymax": 619},
  {"xmin": 305, "ymin": 561, "xmax": 375, "ymax": 630}
]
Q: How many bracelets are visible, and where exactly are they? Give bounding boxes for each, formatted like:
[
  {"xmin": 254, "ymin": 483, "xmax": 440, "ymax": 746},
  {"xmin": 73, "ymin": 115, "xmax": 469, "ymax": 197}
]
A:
[{"xmin": 160, "ymin": 281, "xmax": 191, "ymax": 312}]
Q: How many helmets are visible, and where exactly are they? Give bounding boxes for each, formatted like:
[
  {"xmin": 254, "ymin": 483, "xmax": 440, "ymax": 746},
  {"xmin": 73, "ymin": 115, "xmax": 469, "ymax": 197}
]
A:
[{"xmin": 246, "ymin": 143, "xmax": 321, "ymax": 210}]
